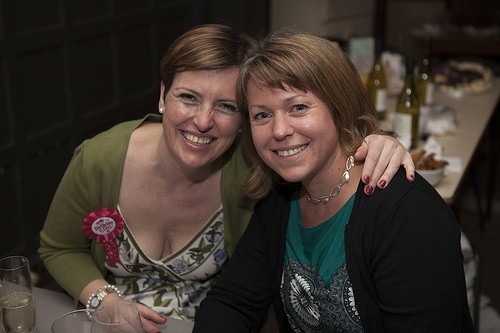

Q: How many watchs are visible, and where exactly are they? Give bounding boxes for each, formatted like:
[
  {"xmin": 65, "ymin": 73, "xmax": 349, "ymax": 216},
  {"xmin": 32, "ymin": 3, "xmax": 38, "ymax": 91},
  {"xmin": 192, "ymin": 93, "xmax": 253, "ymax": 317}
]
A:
[{"xmin": 88, "ymin": 286, "xmax": 116, "ymax": 315}]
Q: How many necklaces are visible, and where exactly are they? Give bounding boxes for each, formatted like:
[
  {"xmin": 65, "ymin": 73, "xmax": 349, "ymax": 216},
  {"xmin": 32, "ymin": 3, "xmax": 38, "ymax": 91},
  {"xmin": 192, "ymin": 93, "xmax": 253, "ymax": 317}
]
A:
[{"xmin": 299, "ymin": 153, "xmax": 359, "ymax": 207}]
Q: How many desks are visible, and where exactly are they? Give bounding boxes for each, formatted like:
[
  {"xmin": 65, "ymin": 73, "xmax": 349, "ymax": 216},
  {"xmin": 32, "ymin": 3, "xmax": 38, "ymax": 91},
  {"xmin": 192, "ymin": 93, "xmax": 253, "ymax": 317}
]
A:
[
  {"xmin": 0, "ymin": 281, "xmax": 194, "ymax": 333},
  {"xmin": 365, "ymin": 62, "xmax": 497, "ymax": 206}
]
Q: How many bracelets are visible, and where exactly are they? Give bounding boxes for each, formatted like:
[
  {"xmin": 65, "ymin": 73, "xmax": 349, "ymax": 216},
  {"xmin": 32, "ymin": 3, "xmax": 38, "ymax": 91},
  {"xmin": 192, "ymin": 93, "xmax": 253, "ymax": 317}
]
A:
[{"xmin": 85, "ymin": 284, "xmax": 121, "ymax": 321}]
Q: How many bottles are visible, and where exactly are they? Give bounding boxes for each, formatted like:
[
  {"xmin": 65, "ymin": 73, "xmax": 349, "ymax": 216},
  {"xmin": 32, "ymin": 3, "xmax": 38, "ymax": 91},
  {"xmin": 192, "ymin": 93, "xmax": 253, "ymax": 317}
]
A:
[
  {"xmin": 414, "ymin": 36, "xmax": 436, "ymax": 107},
  {"xmin": 367, "ymin": 43, "xmax": 386, "ymax": 120},
  {"xmin": 395, "ymin": 59, "xmax": 420, "ymax": 150}
]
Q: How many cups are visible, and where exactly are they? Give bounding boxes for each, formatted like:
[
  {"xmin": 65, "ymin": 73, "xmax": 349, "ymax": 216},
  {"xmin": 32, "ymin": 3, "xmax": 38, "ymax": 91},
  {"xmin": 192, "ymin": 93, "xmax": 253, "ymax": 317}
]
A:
[
  {"xmin": 51, "ymin": 309, "xmax": 112, "ymax": 333},
  {"xmin": 91, "ymin": 299, "xmax": 144, "ymax": 333},
  {"xmin": 0, "ymin": 256, "xmax": 36, "ymax": 333}
]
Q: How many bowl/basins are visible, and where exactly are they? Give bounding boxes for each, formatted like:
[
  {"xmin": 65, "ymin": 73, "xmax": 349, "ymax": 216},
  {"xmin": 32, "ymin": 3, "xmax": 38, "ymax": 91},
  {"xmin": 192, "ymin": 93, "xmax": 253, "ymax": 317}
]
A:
[{"xmin": 415, "ymin": 169, "xmax": 444, "ymax": 187}]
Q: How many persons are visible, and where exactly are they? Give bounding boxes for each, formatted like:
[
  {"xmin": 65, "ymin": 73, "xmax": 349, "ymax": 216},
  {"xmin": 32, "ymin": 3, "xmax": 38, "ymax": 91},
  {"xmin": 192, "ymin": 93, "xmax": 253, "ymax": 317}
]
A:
[
  {"xmin": 192, "ymin": 31, "xmax": 467, "ymax": 333},
  {"xmin": 37, "ymin": 24, "xmax": 416, "ymax": 333}
]
